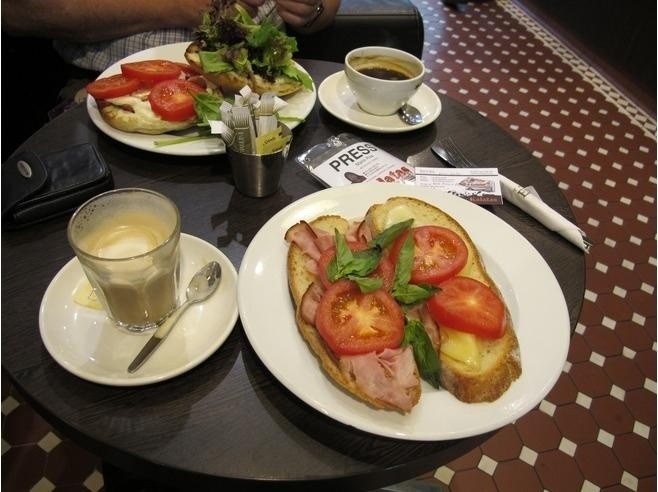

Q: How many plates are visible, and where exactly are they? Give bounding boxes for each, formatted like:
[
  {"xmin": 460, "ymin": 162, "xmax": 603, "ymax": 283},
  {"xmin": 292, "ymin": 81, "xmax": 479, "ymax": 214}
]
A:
[
  {"xmin": 317, "ymin": 67, "xmax": 443, "ymax": 134},
  {"xmin": 86, "ymin": 40, "xmax": 317, "ymax": 158},
  {"xmin": 38, "ymin": 231, "xmax": 238, "ymax": 390},
  {"xmin": 238, "ymin": 183, "xmax": 571, "ymax": 442}
]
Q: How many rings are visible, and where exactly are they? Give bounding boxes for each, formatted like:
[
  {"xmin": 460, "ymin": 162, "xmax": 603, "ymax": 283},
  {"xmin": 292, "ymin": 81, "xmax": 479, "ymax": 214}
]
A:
[{"xmin": 313, "ymin": 1, "xmax": 324, "ymax": 15}]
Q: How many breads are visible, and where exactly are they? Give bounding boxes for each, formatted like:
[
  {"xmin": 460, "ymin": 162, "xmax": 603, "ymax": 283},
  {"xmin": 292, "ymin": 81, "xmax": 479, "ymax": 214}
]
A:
[
  {"xmin": 288, "ymin": 214, "xmax": 422, "ymax": 416},
  {"xmin": 96, "ymin": 70, "xmax": 224, "ymax": 134},
  {"xmin": 184, "ymin": 40, "xmax": 305, "ymax": 98},
  {"xmin": 365, "ymin": 196, "xmax": 522, "ymax": 405}
]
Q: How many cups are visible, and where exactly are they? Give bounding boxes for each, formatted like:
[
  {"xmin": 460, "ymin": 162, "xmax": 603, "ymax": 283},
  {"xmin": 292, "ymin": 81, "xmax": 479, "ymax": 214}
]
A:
[
  {"xmin": 66, "ymin": 187, "xmax": 180, "ymax": 334},
  {"xmin": 345, "ymin": 46, "xmax": 425, "ymax": 116}
]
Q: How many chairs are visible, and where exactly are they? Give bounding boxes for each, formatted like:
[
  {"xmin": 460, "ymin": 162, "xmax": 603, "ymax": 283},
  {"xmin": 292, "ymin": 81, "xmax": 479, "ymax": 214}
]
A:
[{"xmin": 285, "ymin": 1, "xmax": 425, "ymax": 66}]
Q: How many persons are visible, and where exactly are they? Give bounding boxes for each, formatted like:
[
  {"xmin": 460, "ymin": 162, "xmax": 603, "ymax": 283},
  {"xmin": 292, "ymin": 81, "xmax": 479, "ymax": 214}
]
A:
[{"xmin": 2, "ymin": 0, "xmax": 344, "ymax": 153}]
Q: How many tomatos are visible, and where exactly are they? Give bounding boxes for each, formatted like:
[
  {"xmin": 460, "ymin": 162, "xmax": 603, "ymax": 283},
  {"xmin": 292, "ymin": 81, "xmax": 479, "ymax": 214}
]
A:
[{"xmin": 85, "ymin": 58, "xmax": 206, "ymax": 121}]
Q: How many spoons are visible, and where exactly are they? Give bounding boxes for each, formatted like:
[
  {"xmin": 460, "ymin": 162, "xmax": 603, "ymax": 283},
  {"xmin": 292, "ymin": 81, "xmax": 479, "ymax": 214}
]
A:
[{"xmin": 126, "ymin": 260, "xmax": 222, "ymax": 374}]
